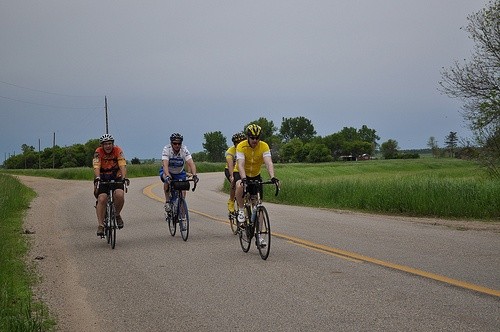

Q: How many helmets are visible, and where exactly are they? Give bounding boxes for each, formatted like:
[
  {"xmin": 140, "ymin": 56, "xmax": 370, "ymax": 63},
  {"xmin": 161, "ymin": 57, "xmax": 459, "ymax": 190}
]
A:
[
  {"xmin": 170, "ymin": 133, "xmax": 183, "ymax": 141},
  {"xmin": 231, "ymin": 132, "xmax": 247, "ymax": 141},
  {"xmin": 246, "ymin": 123, "xmax": 261, "ymax": 136},
  {"xmin": 99, "ymin": 134, "xmax": 114, "ymax": 144}
]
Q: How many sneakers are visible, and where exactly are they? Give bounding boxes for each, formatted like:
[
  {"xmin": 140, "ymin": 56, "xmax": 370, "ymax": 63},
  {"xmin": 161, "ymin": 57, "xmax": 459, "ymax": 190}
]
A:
[
  {"xmin": 255, "ymin": 238, "xmax": 267, "ymax": 245},
  {"xmin": 238, "ymin": 208, "xmax": 245, "ymax": 222},
  {"xmin": 227, "ymin": 199, "xmax": 235, "ymax": 213}
]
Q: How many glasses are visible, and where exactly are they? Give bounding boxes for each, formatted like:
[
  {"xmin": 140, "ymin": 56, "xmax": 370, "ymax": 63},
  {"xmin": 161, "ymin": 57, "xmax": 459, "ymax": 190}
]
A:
[
  {"xmin": 172, "ymin": 142, "xmax": 182, "ymax": 145},
  {"xmin": 249, "ymin": 136, "xmax": 259, "ymax": 140}
]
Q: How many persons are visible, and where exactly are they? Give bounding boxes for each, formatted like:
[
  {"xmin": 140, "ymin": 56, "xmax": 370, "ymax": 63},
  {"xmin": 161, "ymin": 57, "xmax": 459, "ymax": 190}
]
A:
[
  {"xmin": 233, "ymin": 124, "xmax": 280, "ymax": 246},
  {"xmin": 224, "ymin": 133, "xmax": 249, "ymax": 225},
  {"xmin": 92, "ymin": 134, "xmax": 126, "ymax": 236},
  {"xmin": 159, "ymin": 133, "xmax": 196, "ymax": 231}
]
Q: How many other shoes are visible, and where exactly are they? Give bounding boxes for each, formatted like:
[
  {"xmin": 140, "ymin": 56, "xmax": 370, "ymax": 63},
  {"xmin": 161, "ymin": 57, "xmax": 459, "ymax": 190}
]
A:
[
  {"xmin": 164, "ymin": 203, "xmax": 171, "ymax": 213},
  {"xmin": 97, "ymin": 225, "xmax": 104, "ymax": 236},
  {"xmin": 115, "ymin": 215, "xmax": 124, "ymax": 228},
  {"xmin": 181, "ymin": 209, "xmax": 186, "ymax": 221}
]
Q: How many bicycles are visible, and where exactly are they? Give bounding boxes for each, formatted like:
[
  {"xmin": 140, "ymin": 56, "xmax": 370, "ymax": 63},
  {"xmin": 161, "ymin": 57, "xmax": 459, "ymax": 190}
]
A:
[
  {"xmin": 164, "ymin": 175, "xmax": 198, "ymax": 241},
  {"xmin": 226, "ymin": 179, "xmax": 281, "ymax": 260},
  {"xmin": 99, "ymin": 179, "xmax": 128, "ymax": 249}
]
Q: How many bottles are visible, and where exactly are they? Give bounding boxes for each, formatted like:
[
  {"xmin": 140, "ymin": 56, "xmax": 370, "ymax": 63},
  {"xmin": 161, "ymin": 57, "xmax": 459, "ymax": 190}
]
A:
[
  {"xmin": 175, "ymin": 197, "xmax": 179, "ymax": 205},
  {"xmin": 107, "ymin": 199, "xmax": 110, "ymax": 213},
  {"xmin": 251, "ymin": 205, "xmax": 257, "ymax": 223}
]
